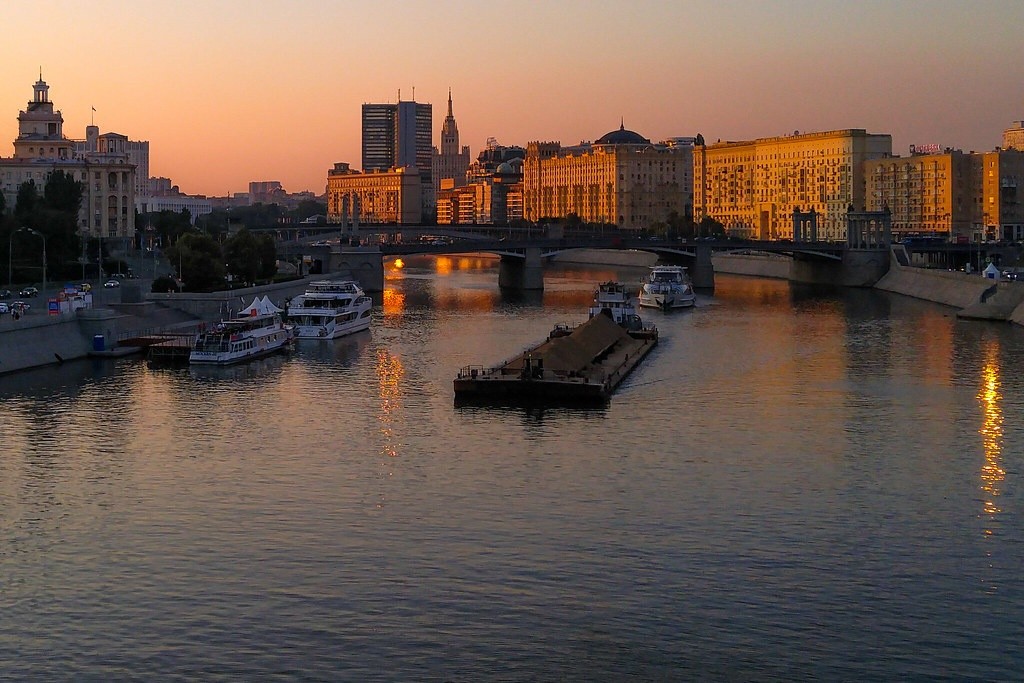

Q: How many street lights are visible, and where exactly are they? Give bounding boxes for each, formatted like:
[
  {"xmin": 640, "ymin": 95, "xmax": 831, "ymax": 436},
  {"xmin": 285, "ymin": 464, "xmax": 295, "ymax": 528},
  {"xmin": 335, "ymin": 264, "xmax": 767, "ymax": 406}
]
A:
[
  {"xmin": 27, "ymin": 229, "xmax": 45, "ymax": 294},
  {"xmin": 9, "ymin": 226, "xmax": 26, "ymax": 287}
]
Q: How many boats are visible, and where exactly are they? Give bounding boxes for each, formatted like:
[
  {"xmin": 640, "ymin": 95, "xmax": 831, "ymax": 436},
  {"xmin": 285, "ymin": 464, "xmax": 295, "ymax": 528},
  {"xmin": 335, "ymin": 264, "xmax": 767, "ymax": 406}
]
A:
[
  {"xmin": 189, "ymin": 295, "xmax": 292, "ymax": 365},
  {"xmin": 589, "ymin": 281, "xmax": 635, "ymax": 325},
  {"xmin": 285, "ymin": 280, "xmax": 373, "ymax": 340},
  {"xmin": 639, "ymin": 264, "xmax": 696, "ymax": 309}
]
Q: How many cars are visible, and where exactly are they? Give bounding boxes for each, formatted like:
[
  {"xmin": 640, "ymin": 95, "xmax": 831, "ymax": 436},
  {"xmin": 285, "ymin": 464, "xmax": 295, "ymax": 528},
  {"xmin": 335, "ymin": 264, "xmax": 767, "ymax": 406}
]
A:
[
  {"xmin": 104, "ymin": 280, "xmax": 120, "ymax": 288},
  {"xmin": 898, "ymin": 240, "xmax": 912, "ymax": 246},
  {"xmin": 0, "ymin": 303, "xmax": 10, "ymax": 313},
  {"xmin": 75, "ymin": 285, "xmax": 82, "ymax": 292},
  {"xmin": 111, "ymin": 272, "xmax": 125, "ymax": 279},
  {"xmin": 961, "ymin": 266, "xmax": 974, "ymax": 271},
  {"xmin": 0, "ymin": 287, "xmax": 12, "ymax": 299},
  {"xmin": 81, "ymin": 284, "xmax": 91, "ymax": 292},
  {"xmin": 705, "ymin": 237, "xmax": 716, "ymax": 242},
  {"xmin": 649, "ymin": 237, "xmax": 661, "ymax": 242},
  {"xmin": 1002, "ymin": 270, "xmax": 1012, "ymax": 275},
  {"xmin": 19, "ymin": 286, "xmax": 39, "ymax": 297},
  {"xmin": 8, "ymin": 299, "xmax": 31, "ymax": 310}
]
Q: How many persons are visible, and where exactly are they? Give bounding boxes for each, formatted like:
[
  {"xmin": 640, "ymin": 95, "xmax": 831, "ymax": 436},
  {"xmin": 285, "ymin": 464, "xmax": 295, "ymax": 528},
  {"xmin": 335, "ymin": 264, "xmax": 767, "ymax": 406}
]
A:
[
  {"xmin": 15, "ymin": 312, "xmax": 20, "ymax": 320},
  {"xmin": 11, "ymin": 307, "xmax": 15, "ymax": 320},
  {"xmin": 19, "ymin": 303, "xmax": 25, "ymax": 319}
]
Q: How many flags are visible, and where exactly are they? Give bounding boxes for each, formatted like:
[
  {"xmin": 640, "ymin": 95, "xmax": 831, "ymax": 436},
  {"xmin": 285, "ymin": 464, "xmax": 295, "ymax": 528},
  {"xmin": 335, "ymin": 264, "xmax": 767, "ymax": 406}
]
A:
[{"xmin": 92, "ymin": 106, "xmax": 96, "ymax": 111}]
